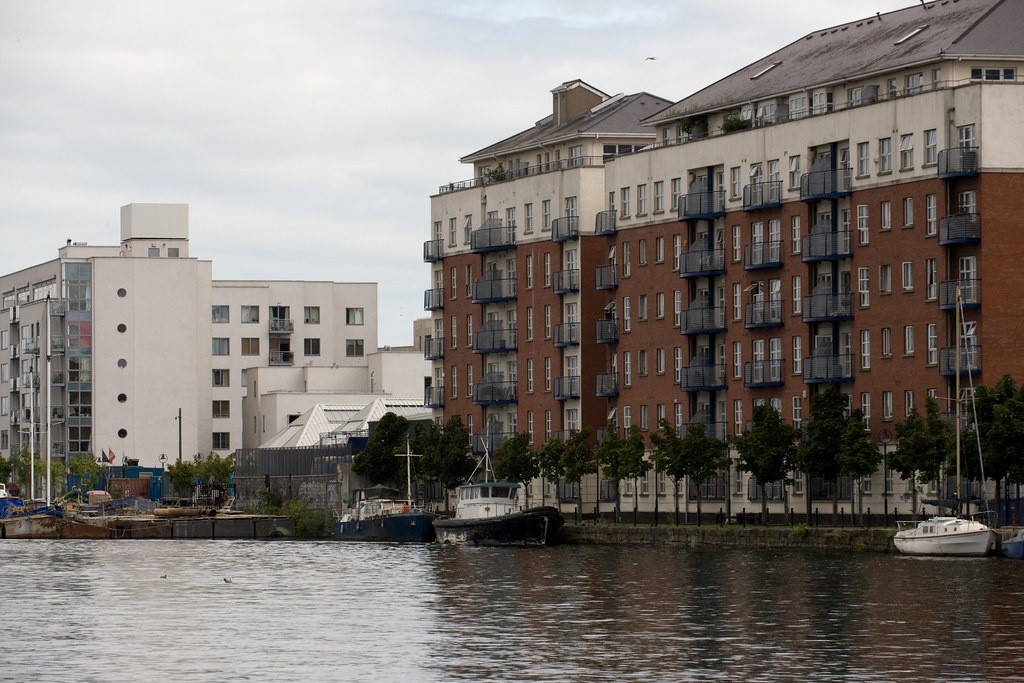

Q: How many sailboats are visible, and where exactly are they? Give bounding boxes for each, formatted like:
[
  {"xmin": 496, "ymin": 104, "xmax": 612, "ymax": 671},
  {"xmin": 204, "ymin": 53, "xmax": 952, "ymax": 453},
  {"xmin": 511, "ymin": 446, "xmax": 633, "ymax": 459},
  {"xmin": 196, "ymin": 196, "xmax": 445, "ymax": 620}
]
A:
[{"xmin": 893, "ymin": 282, "xmax": 998, "ymax": 558}]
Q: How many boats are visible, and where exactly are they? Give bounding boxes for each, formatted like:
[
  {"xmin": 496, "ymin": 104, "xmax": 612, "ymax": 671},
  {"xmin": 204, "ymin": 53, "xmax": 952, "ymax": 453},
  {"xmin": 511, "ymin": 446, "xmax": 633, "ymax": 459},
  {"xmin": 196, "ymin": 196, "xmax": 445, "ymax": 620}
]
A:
[
  {"xmin": 431, "ymin": 417, "xmax": 566, "ymax": 548},
  {"xmin": 1001, "ymin": 525, "xmax": 1024, "ymax": 560},
  {"xmin": 332, "ymin": 432, "xmax": 442, "ymax": 546}
]
